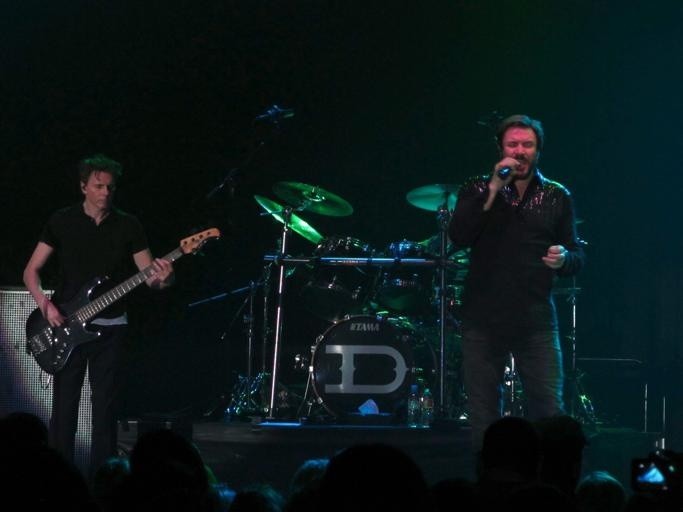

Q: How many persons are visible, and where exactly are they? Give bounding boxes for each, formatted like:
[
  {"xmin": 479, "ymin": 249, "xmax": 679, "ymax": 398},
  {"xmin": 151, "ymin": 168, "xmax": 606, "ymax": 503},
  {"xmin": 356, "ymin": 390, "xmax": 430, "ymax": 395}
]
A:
[
  {"xmin": 573, "ymin": 471, "xmax": 683, "ymax": 511},
  {"xmin": 24, "ymin": 157, "xmax": 176, "ymax": 481},
  {"xmin": 1, "ymin": 412, "xmax": 573, "ymax": 512},
  {"xmin": 449, "ymin": 115, "xmax": 587, "ymax": 441}
]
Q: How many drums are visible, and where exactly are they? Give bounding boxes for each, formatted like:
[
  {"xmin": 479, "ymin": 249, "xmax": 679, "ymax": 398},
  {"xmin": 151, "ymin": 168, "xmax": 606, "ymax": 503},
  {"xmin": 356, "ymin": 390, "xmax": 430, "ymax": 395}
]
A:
[
  {"xmin": 297, "ymin": 237, "xmax": 380, "ymax": 324},
  {"xmin": 376, "ymin": 241, "xmax": 433, "ymax": 315},
  {"xmin": 311, "ymin": 315, "xmax": 436, "ymax": 417}
]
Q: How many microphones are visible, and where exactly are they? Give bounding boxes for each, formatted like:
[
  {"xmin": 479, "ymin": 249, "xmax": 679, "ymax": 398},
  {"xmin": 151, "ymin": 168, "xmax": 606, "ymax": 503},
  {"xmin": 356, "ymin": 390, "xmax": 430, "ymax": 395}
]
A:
[
  {"xmin": 498, "ymin": 162, "xmax": 520, "ymax": 179},
  {"xmin": 257, "ymin": 108, "xmax": 295, "ymax": 122}
]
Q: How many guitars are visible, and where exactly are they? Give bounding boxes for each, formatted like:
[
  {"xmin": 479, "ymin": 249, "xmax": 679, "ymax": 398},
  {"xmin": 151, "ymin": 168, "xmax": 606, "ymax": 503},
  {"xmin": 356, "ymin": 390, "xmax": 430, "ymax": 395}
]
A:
[{"xmin": 26, "ymin": 228, "xmax": 221, "ymax": 374}]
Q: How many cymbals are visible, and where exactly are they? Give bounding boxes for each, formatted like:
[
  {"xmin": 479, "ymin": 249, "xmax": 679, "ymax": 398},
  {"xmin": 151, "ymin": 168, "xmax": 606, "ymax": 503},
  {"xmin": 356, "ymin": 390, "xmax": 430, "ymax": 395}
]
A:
[
  {"xmin": 407, "ymin": 183, "xmax": 462, "ymax": 211},
  {"xmin": 254, "ymin": 182, "xmax": 354, "ymax": 245}
]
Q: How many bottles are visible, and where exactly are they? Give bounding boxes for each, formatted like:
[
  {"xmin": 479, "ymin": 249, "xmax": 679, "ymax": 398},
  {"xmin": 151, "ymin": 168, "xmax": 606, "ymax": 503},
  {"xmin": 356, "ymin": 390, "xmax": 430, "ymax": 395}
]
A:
[{"xmin": 407, "ymin": 384, "xmax": 435, "ymax": 428}]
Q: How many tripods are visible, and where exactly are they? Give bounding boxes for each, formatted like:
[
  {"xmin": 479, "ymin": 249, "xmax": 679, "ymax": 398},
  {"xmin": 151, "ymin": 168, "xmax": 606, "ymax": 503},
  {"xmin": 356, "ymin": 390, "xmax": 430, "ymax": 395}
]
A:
[
  {"xmin": 188, "ymin": 276, "xmax": 268, "ymax": 417},
  {"xmin": 564, "ymin": 284, "xmax": 595, "ymax": 418}
]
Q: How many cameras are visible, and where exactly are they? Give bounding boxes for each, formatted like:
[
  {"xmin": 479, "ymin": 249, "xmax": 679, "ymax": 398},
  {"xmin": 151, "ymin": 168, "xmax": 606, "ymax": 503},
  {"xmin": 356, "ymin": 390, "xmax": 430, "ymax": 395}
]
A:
[{"xmin": 631, "ymin": 457, "xmax": 676, "ymax": 494}]
